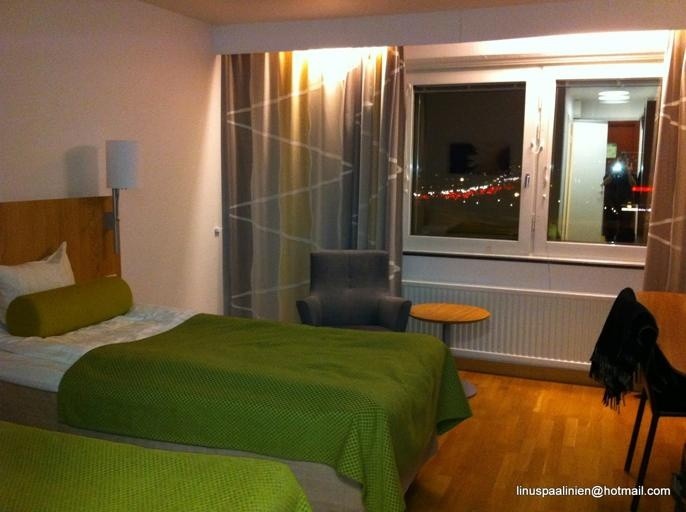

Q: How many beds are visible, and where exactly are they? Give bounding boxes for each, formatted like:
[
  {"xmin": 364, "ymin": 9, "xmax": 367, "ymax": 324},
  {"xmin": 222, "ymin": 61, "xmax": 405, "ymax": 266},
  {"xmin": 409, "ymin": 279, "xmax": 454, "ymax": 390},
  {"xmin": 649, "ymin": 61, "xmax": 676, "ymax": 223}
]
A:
[
  {"xmin": 409, "ymin": 302, "xmax": 491, "ymax": 398},
  {"xmin": 0, "ymin": 194, "xmax": 474, "ymax": 512},
  {"xmin": 0, "ymin": 420, "xmax": 312, "ymax": 511}
]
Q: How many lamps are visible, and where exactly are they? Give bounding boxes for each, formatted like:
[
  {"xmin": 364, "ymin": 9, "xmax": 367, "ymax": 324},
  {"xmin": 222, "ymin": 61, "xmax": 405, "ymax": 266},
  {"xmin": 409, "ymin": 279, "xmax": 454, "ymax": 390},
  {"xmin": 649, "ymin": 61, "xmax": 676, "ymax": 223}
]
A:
[{"xmin": 102, "ymin": 140, "xmax": 139, "ymax": 255}]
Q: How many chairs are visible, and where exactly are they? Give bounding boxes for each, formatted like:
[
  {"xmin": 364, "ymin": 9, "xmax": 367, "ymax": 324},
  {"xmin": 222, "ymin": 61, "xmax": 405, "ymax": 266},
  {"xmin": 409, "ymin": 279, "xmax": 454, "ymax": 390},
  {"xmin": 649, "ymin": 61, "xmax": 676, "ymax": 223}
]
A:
[
  {"xmin": 296, "ymin": 249, "xmax": 413, "ymax": 332},
  {"xmin": 622, "ymin": 286, "xmax": 685, "ymax": 511}
]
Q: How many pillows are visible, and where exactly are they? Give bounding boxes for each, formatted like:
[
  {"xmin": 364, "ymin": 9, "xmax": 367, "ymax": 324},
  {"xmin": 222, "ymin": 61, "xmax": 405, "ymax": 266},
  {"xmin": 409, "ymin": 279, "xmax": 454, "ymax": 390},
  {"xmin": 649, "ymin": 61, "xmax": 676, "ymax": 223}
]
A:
[
  {"xmin": 7, "ymin": 276, "xmax": 134, "ymax": 337},
  {"xmin": 0, "ymin": 240, "xmax": 76, "ymax": 329}
]
